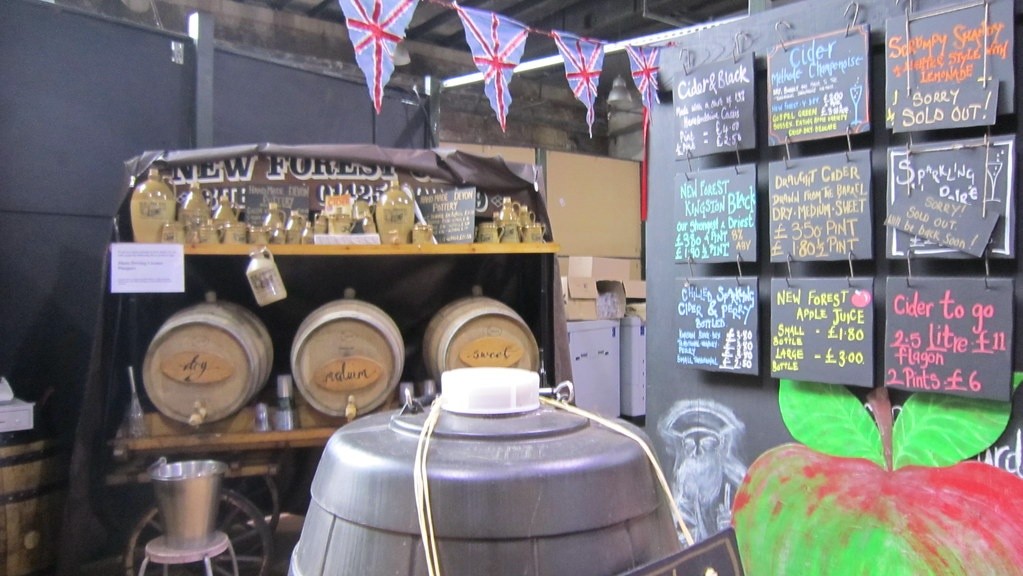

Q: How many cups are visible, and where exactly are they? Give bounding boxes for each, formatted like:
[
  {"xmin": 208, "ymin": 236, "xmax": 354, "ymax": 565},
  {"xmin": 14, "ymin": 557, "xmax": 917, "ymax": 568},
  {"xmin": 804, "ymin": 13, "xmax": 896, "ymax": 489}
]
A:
[
  {"xmin": 161, "ymin": 223, "xmax": 271, "ymax": 246},
  {"xmin": 329, "ymin": 213, "xmax": 354, "ymax": 235},
  {"xmin": 478, "ymin": 222, "xmax": 546, "ymax": 244},
  {"xmin": 412, "ymin": 225, "xmax": 432, "ymax": 244}
]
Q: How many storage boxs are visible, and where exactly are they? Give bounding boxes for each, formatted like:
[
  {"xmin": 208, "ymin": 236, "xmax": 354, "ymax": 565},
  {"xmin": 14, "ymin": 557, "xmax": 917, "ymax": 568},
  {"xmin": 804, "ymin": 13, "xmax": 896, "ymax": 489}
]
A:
[{"xmin": 442, "ymin": 141, "xmax": 646, "ymax": 418}]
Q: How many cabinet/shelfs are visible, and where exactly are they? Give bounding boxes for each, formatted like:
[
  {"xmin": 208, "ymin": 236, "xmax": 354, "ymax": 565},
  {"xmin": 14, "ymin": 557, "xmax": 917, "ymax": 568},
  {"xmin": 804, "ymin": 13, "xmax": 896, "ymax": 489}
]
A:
[{"xmin": 110, "ymin": 238, "xmax": 557, "ymax": 488}]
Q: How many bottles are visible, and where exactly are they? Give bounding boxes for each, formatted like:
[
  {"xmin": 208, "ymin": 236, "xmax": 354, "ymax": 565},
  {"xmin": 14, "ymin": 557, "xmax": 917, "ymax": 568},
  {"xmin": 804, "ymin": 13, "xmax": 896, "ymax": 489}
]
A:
[
  {"xmin": 179, "ymin": 182, "xmax": 238, "ymax": 222},
  {"xmin": 355, "ymin": 179, "xmax": 413, "ymax": 244},
  {"xmin": 263, "ymin": 203, "xmax": 326, "ymax": 244},
  {"xmin": 493, "ymin": 196, "xmax": 536, "ymax": 224},
  {"xmin": 131, "ymin": 169, "xmax": 177, "ymax": 246},
  {"xmin": 399, "ymin": 380, "xmax": 436, "ymax": 408},
  {"xmin": 245, "ymin": 248, "xmax": 287, "ymax": 306},
  {"xmin": 277, "ymin": 376, "xmax": 294, "ymax": 431}
]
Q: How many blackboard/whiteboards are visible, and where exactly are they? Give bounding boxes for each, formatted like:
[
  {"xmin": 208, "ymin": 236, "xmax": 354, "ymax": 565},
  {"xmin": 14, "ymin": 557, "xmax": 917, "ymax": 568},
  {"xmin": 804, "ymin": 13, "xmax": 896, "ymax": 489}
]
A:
[
  {"xmin": 671, "ymin": 1, "xmax": 1015, "ymax": 404},
  {"xmin": 646, "ymin": 0, "xmax": 1023, "ymax": 576}
]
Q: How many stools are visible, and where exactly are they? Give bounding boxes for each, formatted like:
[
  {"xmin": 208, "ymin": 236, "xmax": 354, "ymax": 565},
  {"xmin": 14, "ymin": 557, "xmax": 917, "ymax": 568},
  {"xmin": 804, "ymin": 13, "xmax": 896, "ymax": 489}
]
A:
[{"xmin": 138, "ymin": 532, "xmax": 238, "ymax": 576}]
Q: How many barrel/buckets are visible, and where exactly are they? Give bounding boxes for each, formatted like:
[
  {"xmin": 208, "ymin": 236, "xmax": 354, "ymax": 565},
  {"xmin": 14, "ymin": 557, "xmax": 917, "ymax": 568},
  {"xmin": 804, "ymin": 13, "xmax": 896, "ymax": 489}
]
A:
[
  {"xmin": 290, "ymin": 288, "xmax": 406, "ymax": 419},
  {"xmin": 141, "ymin": 293, "xmax": 273, "ymax": 425},
  {"xmin": 146, "ymin": 454, "xmax": 229, "ymax": 552},
  {"xmin": 277, "ymin": 367, "xmax": 685, "ymax": 576},
  {"xmin": 0, "ymin": 431, "xmax": 48, "ymax": 576},
  {"xmin": 423, "ymin": 287, "xmax": 539, "ymax": 389}
]
